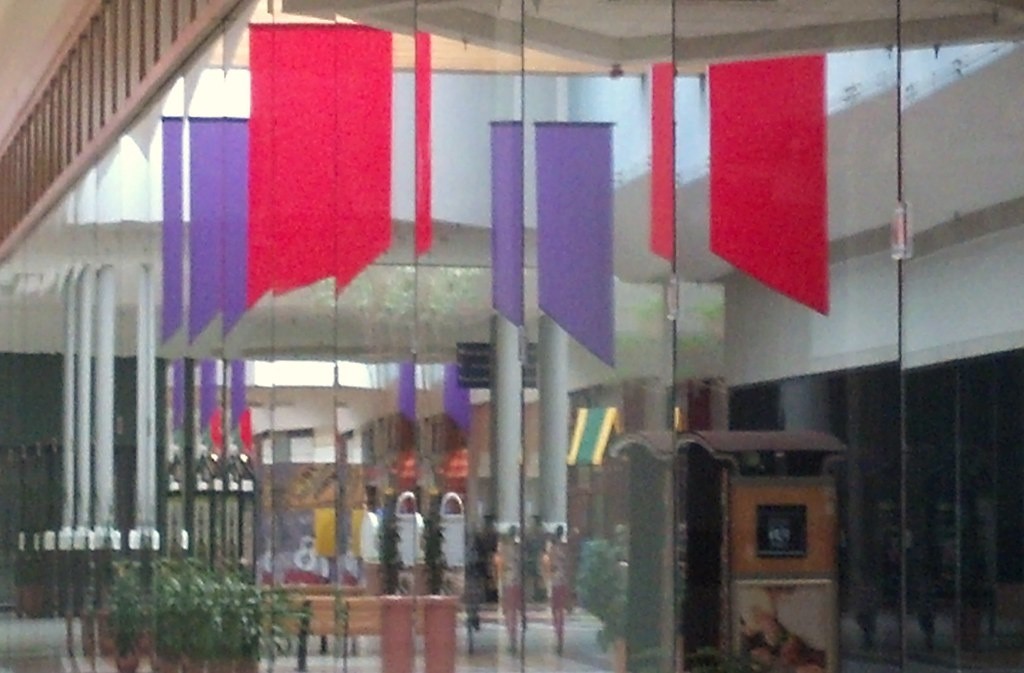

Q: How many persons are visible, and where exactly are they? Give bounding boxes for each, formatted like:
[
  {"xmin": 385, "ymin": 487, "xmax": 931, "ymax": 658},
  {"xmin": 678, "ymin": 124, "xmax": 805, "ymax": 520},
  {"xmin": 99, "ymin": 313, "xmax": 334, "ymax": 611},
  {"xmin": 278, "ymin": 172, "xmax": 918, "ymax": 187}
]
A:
[
  {"xmin": 547, "ymin": 525, "xmax": 569, "ymax": 655},
  {"xmin": 496, "ymin": 525, "xmax": 523, "ymax": 657}
]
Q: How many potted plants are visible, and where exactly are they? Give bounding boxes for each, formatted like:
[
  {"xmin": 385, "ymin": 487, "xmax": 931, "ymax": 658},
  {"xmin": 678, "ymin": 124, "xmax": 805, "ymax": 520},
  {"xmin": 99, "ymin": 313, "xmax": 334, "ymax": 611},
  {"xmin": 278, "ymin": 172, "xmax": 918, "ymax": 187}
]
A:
[
  {"xmin": 377, "ymin": 492, "xmax": 415, "ymax": 672},
  {"xmin": 421, "ymin": 501, "xmax": 456, "ymax": 673},
  {"xmin": 571, "ymin": 518, "xmax": 627, "ymax": 673}
]
